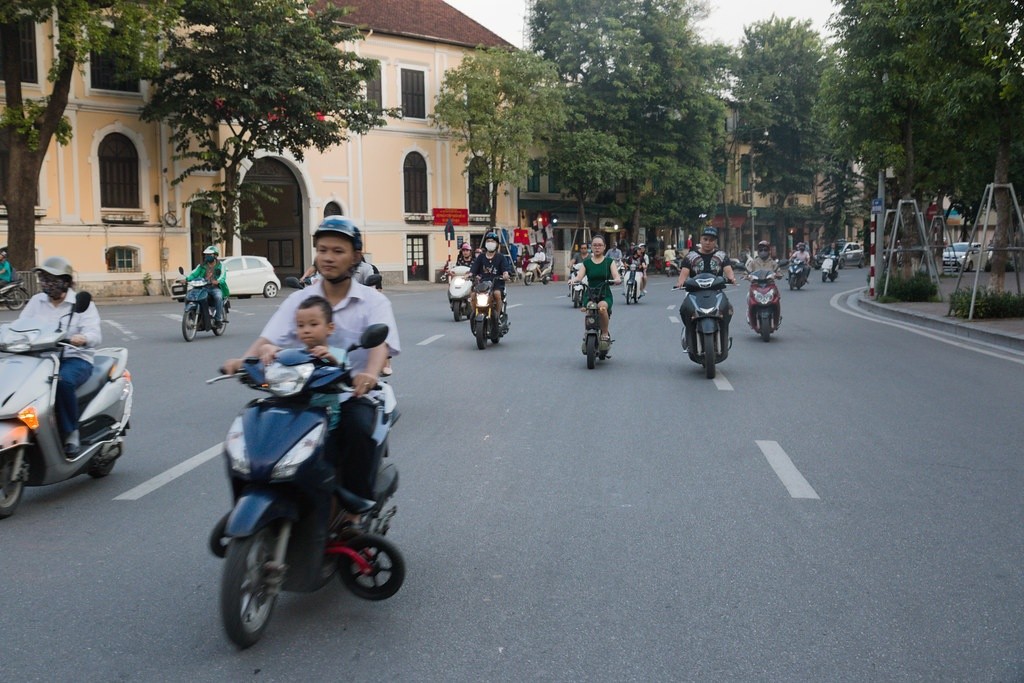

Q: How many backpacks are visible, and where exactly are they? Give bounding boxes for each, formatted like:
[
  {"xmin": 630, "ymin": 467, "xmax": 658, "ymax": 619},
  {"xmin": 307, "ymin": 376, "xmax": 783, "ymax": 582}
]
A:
[{"xmin": 3, "ymin": 259, "xmax": 17, "ymax": 282}]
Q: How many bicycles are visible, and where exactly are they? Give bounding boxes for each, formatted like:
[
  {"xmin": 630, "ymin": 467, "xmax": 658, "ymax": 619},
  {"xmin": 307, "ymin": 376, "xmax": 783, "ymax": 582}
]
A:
[{"xmin": 211, "ymin": 510, "xmax": 406, "ymax": 601}]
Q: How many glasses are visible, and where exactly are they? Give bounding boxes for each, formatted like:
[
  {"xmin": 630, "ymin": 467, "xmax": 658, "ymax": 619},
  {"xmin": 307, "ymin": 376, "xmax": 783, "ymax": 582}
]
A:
[
  {"xmin": 37, "ymin": 273, "xmax": 62, "ymax": 282},
  {"xmin": 759, "ymin": 248, "xmax": 768, "ymax": 251}
]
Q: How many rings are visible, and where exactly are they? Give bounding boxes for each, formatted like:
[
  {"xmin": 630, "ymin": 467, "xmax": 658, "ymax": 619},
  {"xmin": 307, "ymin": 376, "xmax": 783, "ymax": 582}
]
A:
[{"xmin": 364, "ymin": 383, "xmax": 370, "ymax": 385}]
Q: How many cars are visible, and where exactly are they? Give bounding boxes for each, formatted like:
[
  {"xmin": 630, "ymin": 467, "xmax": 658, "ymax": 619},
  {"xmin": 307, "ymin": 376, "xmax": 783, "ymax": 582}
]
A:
[
  {"xmin": 812, "ymin": 241, "xmax": 867, "ymax": 270},
  {"xmin": 942, "ymin": 242, "xmax": 988, "ymax": 272},
  {"xmin": 169, "ymin": 255, "xmax": 281, "ymax": 302}
]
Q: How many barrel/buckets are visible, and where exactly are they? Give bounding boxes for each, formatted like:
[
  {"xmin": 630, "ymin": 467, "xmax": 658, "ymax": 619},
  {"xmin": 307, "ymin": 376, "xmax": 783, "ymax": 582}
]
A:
[{"xmin": 553, "ymin": 275, "xmax": 558, "ymax": 281}]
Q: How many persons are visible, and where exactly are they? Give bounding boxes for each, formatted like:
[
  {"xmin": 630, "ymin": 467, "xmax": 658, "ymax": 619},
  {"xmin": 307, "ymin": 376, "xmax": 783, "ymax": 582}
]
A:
[
  {"xmin": 678, "ymin": 227, "xmax": 737, "ymax": 351},
  {"xmin": 180, "ymin": 246, "xmax": 229, "ymax": 327},
  {"xmin": 567, "ymin": 236, "xmax": 649, "ymax": 342},
  {"xmin": 10, "ymin": 258, "xmax": 102, "ymax": 458},
  {"xmin": 688, "ymin": 234, "xmax": 693, "ymax": 249},
  {"xmin": 300, "ymin": 256, "xmax": 393, "ymax": 375},
  {"xmin": 664, "ymin": 244, "xmax": 685, "ymax": 271},
  {"xmin": 789, "ymin": 243, "xmax": 841, "ymax": 282},
  {"xmin": 528, "ymin": 245, "xmax": 546, "ymax": 277},
  {"xmin": 258, "ymin": 294, "xmax": 348, "ymax": 524},
  {"xmin": 458, "ymin": 232, "xmax": 511, "ymax": 327},
  {"xmin": 223, "ymin": 217, "xmax": 403, "ymax": 497},
  {"xmin": 0, "ymin": 251, "xmax": 10, "ymax": 284},
  {"xmin": 742, "ymin": 242, "xmax": 783, "ymax": 321}
]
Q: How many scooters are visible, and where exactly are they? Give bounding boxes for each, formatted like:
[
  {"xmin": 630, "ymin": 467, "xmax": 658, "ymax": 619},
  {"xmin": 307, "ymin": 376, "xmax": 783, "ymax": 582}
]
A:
[
  {"xmin": 567, "ymin": 263, "xmax": 588, "ymax": 308},
  {"xmin": 524, "ymin": 258, "xmax": 551, "ymax": 286},
  {"xmin": 611, "ymin": 258, "xmax": 683, "ymax": 305},
  {"xmin": 671, "ymin": 273, "xmax": 734, "ymax": 379},
  {"xmin": 0, "ymin": 279, "xmax": 29, "ymax": 310},
  {"xmin": 465, "ymin": 273, "xmax": 511, "ymax": 350},
  {"xmin": 568, "ymin": 280, "xmax": 621, "ymax": 369},
  {"xmin": 735, "ymin": 259, "xmax": 790, "ymax": 343},
  {"xmin": 446, "ymin": 266, "xmax": 474, "ymax": 322},
  {"xmin": 820, "ymin": 254, "xmax": 839, "ymax": 282},
  {"xmin": 206, "ymin": 323, "xmax": 399, "ymax": 648},
  {"xmin": 786, "ymin": 256, "xmax": 811, "ymax": 290},
  {"xmin": 0, "ymin": 291, "xmax": 133, "ymax": 518}
]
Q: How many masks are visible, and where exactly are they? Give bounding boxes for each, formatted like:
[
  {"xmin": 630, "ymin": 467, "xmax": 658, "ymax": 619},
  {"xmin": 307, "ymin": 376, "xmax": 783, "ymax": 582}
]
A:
[
  {"xmin": 759, "ymin": 252, "xmax": 769, "ymax": 258},
  {"xmin": 486, "ymin": 242, "xmax": 497, "ymax": 251},
  {"xmin": 205, "ymin": 255, "xmax": 216, "ymax": 262}
]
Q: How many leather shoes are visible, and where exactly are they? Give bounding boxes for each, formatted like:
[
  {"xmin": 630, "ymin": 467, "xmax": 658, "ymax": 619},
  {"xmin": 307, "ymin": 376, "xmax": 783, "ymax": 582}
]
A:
[{"xmin": 65, "ymin": 431, "xmax": 82, "ymax": 457}]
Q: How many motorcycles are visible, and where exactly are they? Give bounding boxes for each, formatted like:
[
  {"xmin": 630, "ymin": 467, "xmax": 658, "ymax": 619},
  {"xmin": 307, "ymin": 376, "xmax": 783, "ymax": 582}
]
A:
[{"xmin": 175, "ymin": 267, "xmax": 231, "ymax": 342}]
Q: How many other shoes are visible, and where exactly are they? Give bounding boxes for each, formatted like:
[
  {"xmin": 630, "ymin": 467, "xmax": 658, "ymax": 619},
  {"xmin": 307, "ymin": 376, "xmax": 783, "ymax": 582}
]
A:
[
  {"xmin": 214, "ymin": 316, "xmax": 223, "ymax": 329},
  {"xmin": 683, "ymin": 346, "xmax": 689, "ymax": 353},
  {"xmin": 339, "ymin": 520, "xmax": 364, "ymax": 542},
  {"xmin": 600, "ymin": 335, "xmax": 610, "ymax": 341},
  {"xmin": 643, "ymin": 288, "xmax": 647, "ymax": 293},
  {"xmin": 497, "ymin": 317, "xmax": 502, "ymax": 326}
]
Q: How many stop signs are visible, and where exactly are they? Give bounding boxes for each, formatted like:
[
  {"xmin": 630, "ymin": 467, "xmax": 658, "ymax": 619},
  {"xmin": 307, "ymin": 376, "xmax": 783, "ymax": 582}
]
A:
[{"xmin": 928, "ymin": 205, "xmax": 939, "ymax": 221}]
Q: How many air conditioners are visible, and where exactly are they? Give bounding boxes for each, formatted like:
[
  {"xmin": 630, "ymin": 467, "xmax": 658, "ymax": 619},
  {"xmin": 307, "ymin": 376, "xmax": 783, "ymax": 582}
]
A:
[
  {"xmin": 744, "ymin": 192, "xmax": 756, "ymax": 204},
  {"xmin": 770, "ymin": 194, "xmax": 781, "ymax": 205},
  {"xmin": 790, "ymin": 197, "xmax": 801, "ymax": 205}
]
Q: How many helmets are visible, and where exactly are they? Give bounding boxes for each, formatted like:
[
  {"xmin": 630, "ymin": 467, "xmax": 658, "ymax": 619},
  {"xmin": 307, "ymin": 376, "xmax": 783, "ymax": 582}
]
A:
[
  {"xmin": 31, "ymin": 256, "xmax": 72, "ymax": 277},
  {"xmin": 633, "ymin": 245, "xmax": 640, "ymax": 251},
  {"xmin": 797, "ymin": 242, "xmax": 806, "ymax": 249},
  {"xmin": 758, "ymin": 240, "xmax": 770, "ymax": 248},
  {"xmin": 484, "ymin": 232, "xmax": 498, "ymax": 243},
  {"xmin": 701, "ymin": 227, "xmax": 720, "ymax": 238},
  {"xmin": 639, "ymin": 243, "xmax": 645, "ymax": 247},
  {"xmin": 462, "ymin": 243, "xmax": 471, "ymax": 250},
  {"xmin": 312, "ymin": 215, "xmax": 362, "ymax": 251},
  {"xmin": 538, "ymin": 244, "xmax": 543, "ymax": 250},
  {"xmin": 203, "ymin": 245, "xmax": 219, "ymax": 256}
]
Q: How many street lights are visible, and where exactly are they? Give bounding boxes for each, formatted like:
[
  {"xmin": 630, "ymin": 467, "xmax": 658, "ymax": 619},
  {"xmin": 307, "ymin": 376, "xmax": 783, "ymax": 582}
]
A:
[{"xmin": 750, "ymin": 127, "xmax": 769, "ymax": 259}]
